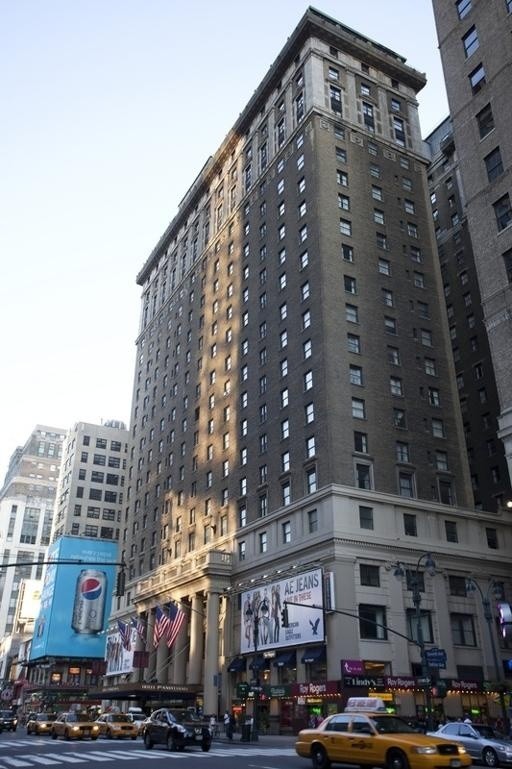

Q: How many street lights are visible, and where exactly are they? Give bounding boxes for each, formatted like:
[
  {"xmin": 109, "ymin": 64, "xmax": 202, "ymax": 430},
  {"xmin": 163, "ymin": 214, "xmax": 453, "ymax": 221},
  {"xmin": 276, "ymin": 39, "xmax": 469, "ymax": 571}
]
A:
[
  {"xmin": 464, "ymin": 574, "xmax": 511, "ymax": 738},
  {"xmin": 392, "ymin": 550, "xmax": 438, "ymax": 734},
  {"xmin": 245, "ymin": 598, "xmax": 269, "ymax": 684}
]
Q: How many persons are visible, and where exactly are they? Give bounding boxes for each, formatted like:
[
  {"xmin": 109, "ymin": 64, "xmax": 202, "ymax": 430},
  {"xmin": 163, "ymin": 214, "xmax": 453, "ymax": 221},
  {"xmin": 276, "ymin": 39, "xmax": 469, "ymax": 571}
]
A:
[
  {"xmin": 307, "ymin": 713, "xmax": 324, "ymax": 729},
  {"xmin": 209, "ymin": 712, "xmax": 216, "ymax": 738},
  {"xmin": 437, "ymin": 712, "xmax": 505, "ymax": 735},
  {"xmin": 105, "ymin": 634, "xmax": 123, "ymax": 672},
  {"xmin": 223, "ymin": 710, "xmax": 230, "ymax": 736},
  {"xmin": 242, "ymin": 585, "xmax": 282, "ymax": 649},
  {"xmin": 227, "ymin": 713, "xmax": 235, "ymax": 740}
]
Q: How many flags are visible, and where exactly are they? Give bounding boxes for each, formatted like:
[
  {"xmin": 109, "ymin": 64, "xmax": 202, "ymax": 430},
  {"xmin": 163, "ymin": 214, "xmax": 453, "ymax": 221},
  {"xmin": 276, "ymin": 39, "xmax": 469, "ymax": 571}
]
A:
[
  {"xmin": 130, "ymin": 617, "xmax": 149, "ymax": 650},
  {"xmin": 152, "ymin": 604, "xmax": 169, "ymax": 650},
  {"xmin": 116, "ymin": 618, "xmax": 131, "ymax": 652},
  {"xmin": 165, "ymin": 600, "xmax": 186, "ymax": 649}
]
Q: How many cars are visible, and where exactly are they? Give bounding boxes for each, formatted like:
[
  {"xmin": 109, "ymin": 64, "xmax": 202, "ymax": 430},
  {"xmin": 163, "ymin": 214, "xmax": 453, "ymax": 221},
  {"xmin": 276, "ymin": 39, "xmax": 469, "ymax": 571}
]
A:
[
  {"xmin": 0, "ymin": 702, "xmax": 217, "ymax": 753},
  {"xmin": 293, "ymin": 697, "xmax": 470, "ymax": 769},
  {"xmin": 428, "ymin": 718, "xmax": 511, "ymax": 768}
]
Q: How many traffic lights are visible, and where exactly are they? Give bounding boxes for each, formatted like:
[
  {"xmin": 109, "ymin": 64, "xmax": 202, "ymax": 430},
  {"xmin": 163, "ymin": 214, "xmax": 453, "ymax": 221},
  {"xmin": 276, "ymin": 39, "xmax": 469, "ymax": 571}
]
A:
[
  {"xmin": 280, "ymin": 608, "xmax": 289, "ymax": 628},
  {"xmin": 430, "ymin": 684, "xmax": 441, "ymax": 698}
]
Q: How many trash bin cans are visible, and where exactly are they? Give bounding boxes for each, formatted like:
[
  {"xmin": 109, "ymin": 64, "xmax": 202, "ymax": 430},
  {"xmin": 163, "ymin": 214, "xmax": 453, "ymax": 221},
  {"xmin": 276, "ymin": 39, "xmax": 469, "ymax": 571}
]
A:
[{"xmin": 266, "ymin": 715, "xmax": 280, "ymax": 735}]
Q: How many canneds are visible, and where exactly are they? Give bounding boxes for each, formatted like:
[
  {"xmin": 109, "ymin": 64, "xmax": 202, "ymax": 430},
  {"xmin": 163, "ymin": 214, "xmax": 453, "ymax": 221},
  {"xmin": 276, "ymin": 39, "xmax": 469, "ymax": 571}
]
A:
[{"xmin": 71, "ymin": 569, "xmax": 108, "ymax": 634}]
